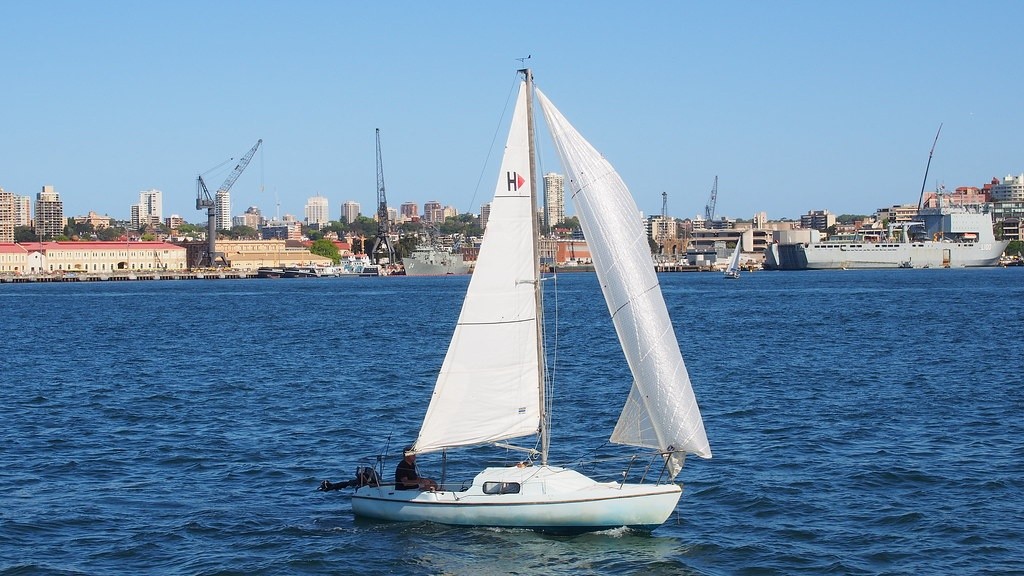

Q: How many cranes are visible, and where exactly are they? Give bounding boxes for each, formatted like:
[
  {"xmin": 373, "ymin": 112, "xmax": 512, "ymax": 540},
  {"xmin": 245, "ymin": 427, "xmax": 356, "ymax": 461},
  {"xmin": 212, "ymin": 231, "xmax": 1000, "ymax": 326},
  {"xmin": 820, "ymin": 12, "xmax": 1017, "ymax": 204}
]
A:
[
  {"xmin": 193, "ymin": 139, "xmax": 266, "ymax": 269},
  {"xmin": 703, "ymin": 175, "xmax": 718, "ymax": 229},
  {"xmin": 370, "ymin": 128, "xmax": 397, "ymax": 267}
]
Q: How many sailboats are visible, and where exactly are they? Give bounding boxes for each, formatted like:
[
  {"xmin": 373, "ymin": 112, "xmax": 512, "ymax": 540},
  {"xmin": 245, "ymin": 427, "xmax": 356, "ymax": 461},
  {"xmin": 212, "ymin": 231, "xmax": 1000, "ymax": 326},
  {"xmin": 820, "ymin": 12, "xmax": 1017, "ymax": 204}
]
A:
[
  {"xmin": 723, "ymin": 236, "xmax": 742, "ymax": 279},
  {"xmin": 312, "ymin": 64, "xmax": 716, "ymax": 528}
]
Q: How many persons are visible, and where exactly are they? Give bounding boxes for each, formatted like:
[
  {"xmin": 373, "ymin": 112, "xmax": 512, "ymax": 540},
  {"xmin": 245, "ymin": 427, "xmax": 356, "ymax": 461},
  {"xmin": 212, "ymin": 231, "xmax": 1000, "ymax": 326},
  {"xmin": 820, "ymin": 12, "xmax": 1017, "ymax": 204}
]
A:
[{"xmin": 395, "ymin": 448, "xmax": 438, "ymax": 492}]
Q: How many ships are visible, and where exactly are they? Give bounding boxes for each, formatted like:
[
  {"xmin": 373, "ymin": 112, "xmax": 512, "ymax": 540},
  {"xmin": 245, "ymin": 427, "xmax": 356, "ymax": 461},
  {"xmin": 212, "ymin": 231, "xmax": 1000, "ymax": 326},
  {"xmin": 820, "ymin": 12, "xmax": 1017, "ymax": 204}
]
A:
[{"xmin": 766, "ymin": 185, "xmax": 1011, "ymax": 270}]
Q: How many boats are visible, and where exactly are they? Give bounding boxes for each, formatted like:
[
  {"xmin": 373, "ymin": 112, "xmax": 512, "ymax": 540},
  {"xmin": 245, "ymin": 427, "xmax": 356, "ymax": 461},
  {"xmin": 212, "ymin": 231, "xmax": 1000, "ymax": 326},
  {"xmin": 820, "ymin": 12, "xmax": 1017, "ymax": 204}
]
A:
[{"xmin": 900, "ymin": 257, "xmax": 914, "ymax": 269}]
